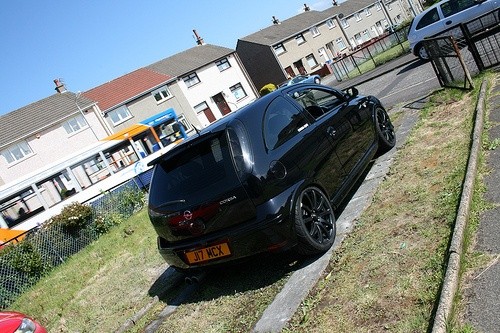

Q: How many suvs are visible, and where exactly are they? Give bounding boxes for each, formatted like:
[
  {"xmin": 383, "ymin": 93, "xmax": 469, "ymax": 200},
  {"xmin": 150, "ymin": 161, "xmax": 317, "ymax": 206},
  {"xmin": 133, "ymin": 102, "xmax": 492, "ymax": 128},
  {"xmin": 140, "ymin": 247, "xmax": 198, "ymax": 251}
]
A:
[{"xmin": 146, "ymin": 83, "xmax": 396, "ymax": 275}]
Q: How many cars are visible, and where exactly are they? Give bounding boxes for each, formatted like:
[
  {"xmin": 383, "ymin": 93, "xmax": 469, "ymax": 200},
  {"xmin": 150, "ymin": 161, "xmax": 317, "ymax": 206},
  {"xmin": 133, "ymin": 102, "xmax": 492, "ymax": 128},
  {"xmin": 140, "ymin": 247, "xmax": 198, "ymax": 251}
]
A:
[
  {"xmin": 277, "ymin": 74, "xmax": 321, "ymax": 85},
  {"xmin": 0, "ymin": 311, "xmax": 47, "ymax": 333},
  {"xmin": 407, "ymin": 0, "xmax": 500, "ymax": 60}
]
